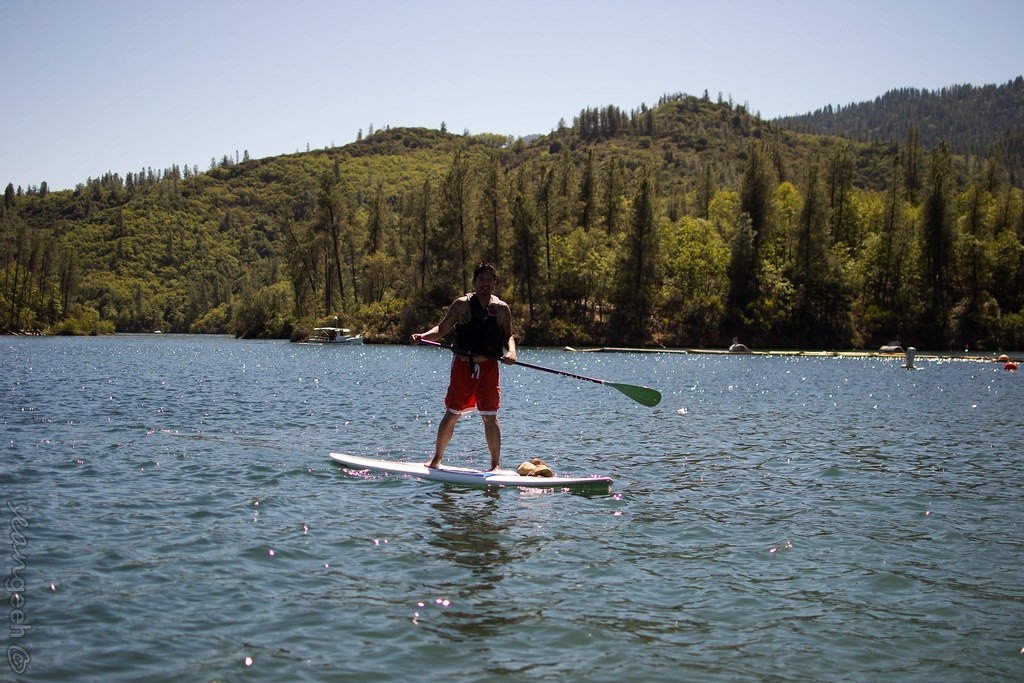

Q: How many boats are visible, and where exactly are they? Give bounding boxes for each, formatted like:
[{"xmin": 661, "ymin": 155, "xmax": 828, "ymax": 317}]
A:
[
  {"xmin": 155, "ymin": 330, "xmax": 161, "ymax": 333},
  {"xmin": 308, "ymin": 327, "xmax": 363, "ymax": 346}
]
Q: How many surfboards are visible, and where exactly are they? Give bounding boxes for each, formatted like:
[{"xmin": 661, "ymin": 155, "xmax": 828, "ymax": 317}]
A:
[{"xmin": 329, "ymin": 452, "xmax": 614, "ymax": 489}]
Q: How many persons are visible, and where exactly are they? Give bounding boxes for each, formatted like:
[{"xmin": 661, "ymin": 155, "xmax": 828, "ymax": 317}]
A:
[{"xmin": 411, "ymin": 262, "xmax": 516, "ymax": 472}]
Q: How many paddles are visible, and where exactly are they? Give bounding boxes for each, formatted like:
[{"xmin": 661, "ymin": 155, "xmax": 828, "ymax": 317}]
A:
[{"xmin": 415, "ymin": 336, "xmax": 662, "ymax": 408}]
